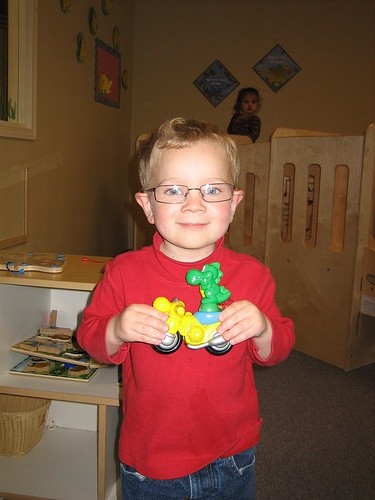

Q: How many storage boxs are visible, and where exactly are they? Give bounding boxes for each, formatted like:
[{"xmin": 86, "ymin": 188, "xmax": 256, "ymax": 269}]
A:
[{"xmin": 0, "ymin": 394, "xmax": 52, "ymax": 458}]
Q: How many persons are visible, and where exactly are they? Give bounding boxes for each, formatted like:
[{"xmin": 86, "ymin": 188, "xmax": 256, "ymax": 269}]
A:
[
  {"xmin": 227, "ymin": 87, "xmax": 261, "ymax": 144},
  {"xmin": 77, "ymin": 117, "xmax": 296, "ymax": 500}
]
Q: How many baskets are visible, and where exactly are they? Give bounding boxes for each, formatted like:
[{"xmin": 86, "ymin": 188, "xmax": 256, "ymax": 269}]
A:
[{"xmin": 0, "ymin": 393, "xmax": 52, "ymax": 458}]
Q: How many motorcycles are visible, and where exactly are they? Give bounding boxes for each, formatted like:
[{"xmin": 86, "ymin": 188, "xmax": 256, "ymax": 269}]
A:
[{"xmin": 150, "ymin": 261, "xmax": 239, "ymax": 355}]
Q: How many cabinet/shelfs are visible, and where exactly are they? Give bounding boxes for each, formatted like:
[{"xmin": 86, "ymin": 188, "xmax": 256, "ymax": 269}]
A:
[{"xmin": 2, "ymin": 251, "xmax": 122, "ymax": 500}]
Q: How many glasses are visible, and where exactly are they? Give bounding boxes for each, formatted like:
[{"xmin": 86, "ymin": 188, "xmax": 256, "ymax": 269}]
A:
[{"xmin": 143, "ymin": 183, "xmax": 237, "ymax": 204}]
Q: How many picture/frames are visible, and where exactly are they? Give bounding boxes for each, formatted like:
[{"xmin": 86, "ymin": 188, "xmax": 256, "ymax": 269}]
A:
[
  {"xmin": 252, "ymin": 44, "xmax": 302, "ymax": 93},
  {"xmin": 94, "ymin": 38, "xmax": 122, "ymax": 109},
  {"xmin": 193, "ymin": 59, "xmax": 240, "ymax": 108}
]
